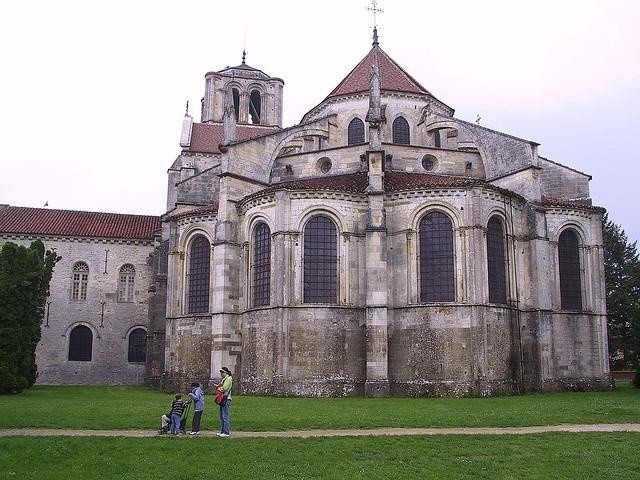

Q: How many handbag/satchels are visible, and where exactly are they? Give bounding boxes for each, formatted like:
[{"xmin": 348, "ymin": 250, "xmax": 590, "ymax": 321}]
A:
[{"xmin": 214, "ymin": 392, "xmax": 227, "ymax": 406}]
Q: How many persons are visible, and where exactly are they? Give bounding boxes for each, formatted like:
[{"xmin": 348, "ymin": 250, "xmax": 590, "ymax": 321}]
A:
[
  {"xmin": 210, "ymin": 367, "xmax": 232, "ymax": 438},
  {"xmin": 158, "ymin": 381, "xmax": 204, "ymax": 436}
]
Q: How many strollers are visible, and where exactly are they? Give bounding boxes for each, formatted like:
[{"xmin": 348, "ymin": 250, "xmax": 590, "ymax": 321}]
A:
[{"xmin": 161, "ymin": 398, "xmax": 192, "ymax": 435}]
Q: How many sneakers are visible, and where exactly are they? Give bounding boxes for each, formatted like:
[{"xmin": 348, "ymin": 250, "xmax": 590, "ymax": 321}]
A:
[
  {"xmin": 216, "ymin": 432, "xmax": 230, "ymax": 437},
  {"xmin": 189, "ymin": 430, "xmax": 200, "ymax": 435}
]
